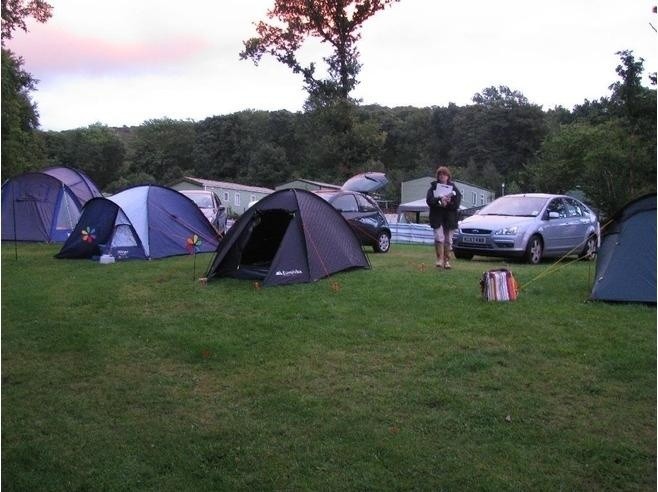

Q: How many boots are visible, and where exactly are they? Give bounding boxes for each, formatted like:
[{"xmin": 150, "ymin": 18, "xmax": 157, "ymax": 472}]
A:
[{"xmin": 435, "ymin": 240, "xmax": 453, "ymax": 269}]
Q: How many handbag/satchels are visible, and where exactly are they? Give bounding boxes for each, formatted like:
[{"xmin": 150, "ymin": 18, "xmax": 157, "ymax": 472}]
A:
[{"xmin": 480, "ymin": 269, "xmax": 519, "ymax": 302}]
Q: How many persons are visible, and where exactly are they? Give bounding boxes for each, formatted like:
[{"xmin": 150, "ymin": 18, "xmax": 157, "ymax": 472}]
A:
[{"xmin": 426, "ymin": 167, "xmax": 461, "ymax": 268}]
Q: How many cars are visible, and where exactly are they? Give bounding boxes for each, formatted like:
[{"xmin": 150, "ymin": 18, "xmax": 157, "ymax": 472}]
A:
[
  {"xmin": 450, "ymin": 193, "xmax": 601, "ymax": 265},
  {"xmin": 313, "ymin": 190, "xmax": 392, "ymax": 253},
  {"xmin": 177, "ymin": 189, "xmax": 228, "ymax": 239}
]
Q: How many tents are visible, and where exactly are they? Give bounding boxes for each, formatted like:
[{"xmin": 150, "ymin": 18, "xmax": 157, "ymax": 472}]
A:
[
  {"xmin": 202, "ymin": 187, "xmax": 370, "ymax": 288},
  {"xmin": 1, "ymin": 166, "xmax": 104, "ymax": 247},
  {"xmin": 52, "ymin": 184, "xmax": 222, "ymax": 262},
  {"xmin": 583, "ymin": 191, "xmax": 657, "ymax": 303}
]
